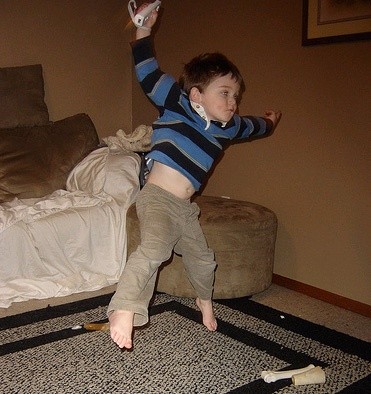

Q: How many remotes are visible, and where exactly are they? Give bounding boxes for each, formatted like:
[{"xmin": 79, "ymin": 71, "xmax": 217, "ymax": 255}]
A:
[{"xmin": 134, "ymin": 0, "xmax": 163, "ymax": 27}]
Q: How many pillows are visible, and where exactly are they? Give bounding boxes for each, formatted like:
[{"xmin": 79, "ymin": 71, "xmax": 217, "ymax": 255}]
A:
[{"xmin": 1, "ymin": 112, "xmax": 99, "ymax": 199}]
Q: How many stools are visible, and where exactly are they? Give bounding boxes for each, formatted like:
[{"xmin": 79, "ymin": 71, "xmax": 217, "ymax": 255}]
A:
[{"xmin": 126, "ymin": 196, "xmax": 276, "ymax": 301}]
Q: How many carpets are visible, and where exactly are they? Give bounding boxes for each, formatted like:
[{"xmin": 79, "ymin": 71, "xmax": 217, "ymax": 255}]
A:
[{"xmin": 0, "ymin": 299, "xmax": 371, "ymax": 393}]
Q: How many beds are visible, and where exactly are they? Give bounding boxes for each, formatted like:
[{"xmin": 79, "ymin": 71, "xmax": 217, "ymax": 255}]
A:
[{"xmin": 1, "ymin": 63, "xmax": 132, "ymax": 293}]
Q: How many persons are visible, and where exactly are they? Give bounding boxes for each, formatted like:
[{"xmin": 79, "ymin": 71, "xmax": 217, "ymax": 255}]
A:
[{"xmin": 106, "ymin": 0, "xmax": 282, "ymax": 349}]
